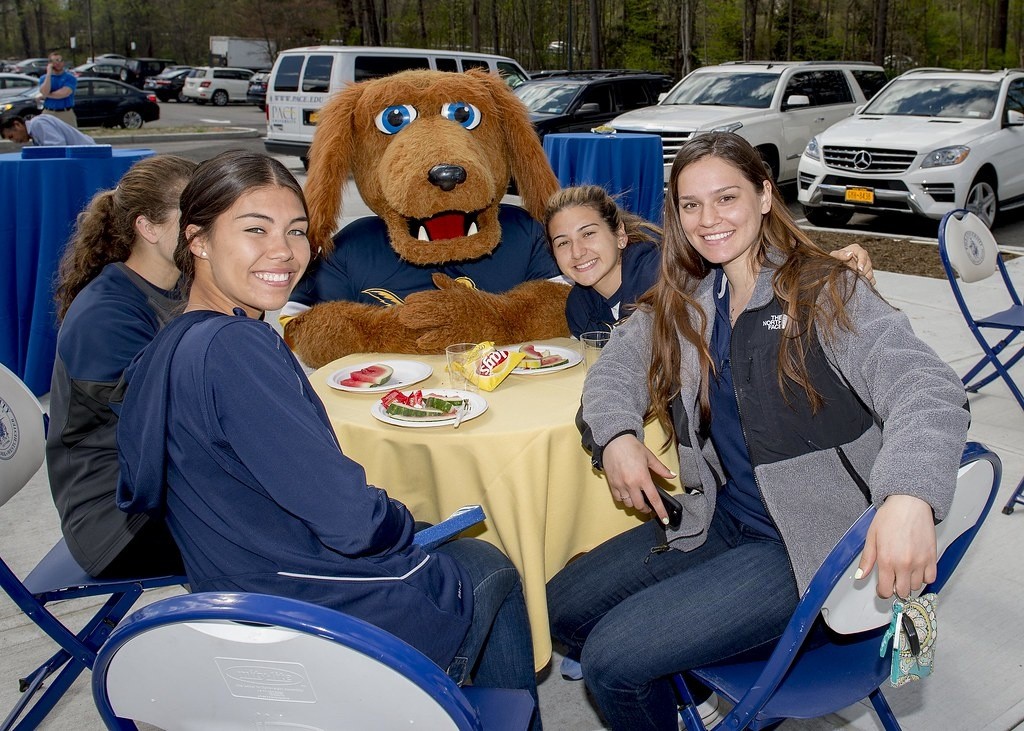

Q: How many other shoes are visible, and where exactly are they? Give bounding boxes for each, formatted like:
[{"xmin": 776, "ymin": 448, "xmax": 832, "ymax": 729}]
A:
[
  {"xmin": 678, "ymin": 691, "xmax": 720, "ymax": 731},
  {"xmin": 559, "ymin": 655, "xmax": 584, "ymax": 681}
]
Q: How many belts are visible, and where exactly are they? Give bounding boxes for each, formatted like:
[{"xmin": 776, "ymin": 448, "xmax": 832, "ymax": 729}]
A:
[{"xmin": 44, "ymin": 105, "xmax": 72, "ymax": 112}]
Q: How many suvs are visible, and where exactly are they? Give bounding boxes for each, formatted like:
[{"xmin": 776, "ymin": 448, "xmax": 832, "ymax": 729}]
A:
[
  {"xmin": 795, "ymin": 68, "xmax": 1024, "ymax": 229},
  {"xmin": 131, "ymin": 57, "xmax": 179, "ymax": 89},
  {"xmin": 182, "ymin": 64, "xmax": 260, "ymax": 108},
  {"xmin": 513, "ymin": 68, "xmax": 690, "ymax": 146},
  {"xmin": 594, "ymin": 61, "xmax": 889, "ymax": 201}
]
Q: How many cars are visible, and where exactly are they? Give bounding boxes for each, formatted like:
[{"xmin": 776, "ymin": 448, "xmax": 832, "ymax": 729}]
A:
[
  {"xmin": 70, "ymin": 62, "xmax": 138, "ymax": 83},
  {"xmin": 0, "ymin": 71, "xmax": 41, "ymax": 103},
  {"xmin": 144, "ymin": 68, "xmax": 193, "ymax": 103},
  {"xmin": 10, "ymin": 58, "xmax": 49, "ymax": 73},
  {"xmin": 86, "ymin": 53, "xmax": 131, "ymax": 65},
  {"xmin": 0, "ymin": 78, "xmax": 160, "ymax": 130}
]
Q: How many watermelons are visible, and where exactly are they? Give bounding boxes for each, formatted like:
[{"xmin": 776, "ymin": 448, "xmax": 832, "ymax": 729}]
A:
[{"xmin": 340, "ymin": 364, "xmax": 394, "ymax": 387}]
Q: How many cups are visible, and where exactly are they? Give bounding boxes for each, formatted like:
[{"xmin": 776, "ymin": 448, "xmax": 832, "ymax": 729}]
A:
[
  {"xmin": 445, "ymin": 343, "xmax": 481, "ymax": 392},
  {"xmin": 579, "ymin": 332, "xmax": 611, "ymax": 378}
]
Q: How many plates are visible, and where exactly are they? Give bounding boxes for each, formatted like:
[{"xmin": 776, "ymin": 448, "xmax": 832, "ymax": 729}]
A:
[
  {"xmin": 326, "ymin": 358, "xmax": 433, "ymax": 394},
  {"xmin": 506, "ymin": 345, "xmax": 583, "ymax": 374},
  {"xmin": 370, "ymin": 388, "xmax": 488, "ymax": 426}
]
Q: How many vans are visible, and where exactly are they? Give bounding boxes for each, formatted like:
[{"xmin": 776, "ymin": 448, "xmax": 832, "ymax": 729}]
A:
[
  {"xmin": 264, "ymin": 44, "xmax": 544, "ymax": 170},
  {"xmin": 248, "ymin": 69, "xmax": 271, "ymax": 110}
]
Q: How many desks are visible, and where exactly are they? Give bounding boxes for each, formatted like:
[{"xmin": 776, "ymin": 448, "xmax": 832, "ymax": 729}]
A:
[
  {"xmin": 0, "ymin": 147, "xmax": 155, "ymax": 399},
  {"xmin": 543, "ymin": 133, "xmax": 664, "ymax": 229}
]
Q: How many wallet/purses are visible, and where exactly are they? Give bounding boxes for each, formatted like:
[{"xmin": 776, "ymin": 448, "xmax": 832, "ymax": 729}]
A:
[{"xmin": 878, "ymin": 593, "xmax": 939, "ymax": 689}]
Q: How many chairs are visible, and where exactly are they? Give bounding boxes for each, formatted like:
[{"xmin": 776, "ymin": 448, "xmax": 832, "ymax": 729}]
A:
[
  {"xmin": 0, "ymin": 361, "xmax": 189, "ymax": 731},
  {"xmin": 90, "ymin": 592, "xmax": 536, "ymax": 731},
  {"xmin": 937, "ymin": 210, "xmax": 1024, "ymax": 411},
  {"xmin": 671, "ymin": 441, "xmax": 1003, "ymax": 731}
]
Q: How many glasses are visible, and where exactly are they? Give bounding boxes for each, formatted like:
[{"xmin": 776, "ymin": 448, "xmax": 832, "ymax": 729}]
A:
[{"xmin": 50, "ymin": 58, "xmax": 64, "ymax": 62}]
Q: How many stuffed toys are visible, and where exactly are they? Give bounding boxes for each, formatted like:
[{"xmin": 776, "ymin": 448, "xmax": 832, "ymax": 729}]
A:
[{"xmin": 279, "ymin": 67, "xmax": 570, "ymax": 369}]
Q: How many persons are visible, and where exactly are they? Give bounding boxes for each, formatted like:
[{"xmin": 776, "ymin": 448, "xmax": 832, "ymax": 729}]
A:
[
  {"xmin": 0, "ymin": 115, "xmax": 97, "ymax": 145},
  {"xmin": 543, "ymin": 184, "xmax": 875, "ymax": 680},
  {"xmin": 546, "ymin": 132, "xmax": 970, "ymax": 731},
  {"xmin": 108, "ymin": 148, "xmax": 543, "ymax": 731},
  {"xmin": 45, "ymin": 156, "xmax": 199, "ymax": 580},
  {"xmin": 39, "ymin": 51, "xmax": 78, "ymax": 129}
]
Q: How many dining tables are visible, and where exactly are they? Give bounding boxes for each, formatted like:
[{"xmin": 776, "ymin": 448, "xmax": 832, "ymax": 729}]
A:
[{"xmin": 307, "ymin": 336, "xmax": 700, "ymax": 674}]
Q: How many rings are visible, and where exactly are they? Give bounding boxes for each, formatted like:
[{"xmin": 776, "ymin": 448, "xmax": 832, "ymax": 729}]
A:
[{"xmin": 621, "ymin": 496, "xmax": 631, "ymax": 500}]
